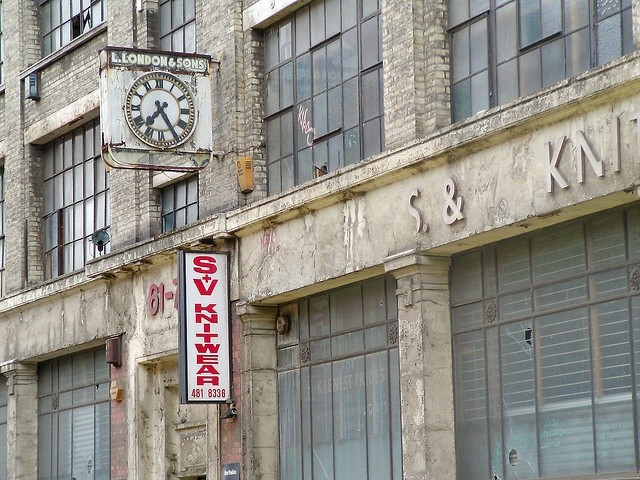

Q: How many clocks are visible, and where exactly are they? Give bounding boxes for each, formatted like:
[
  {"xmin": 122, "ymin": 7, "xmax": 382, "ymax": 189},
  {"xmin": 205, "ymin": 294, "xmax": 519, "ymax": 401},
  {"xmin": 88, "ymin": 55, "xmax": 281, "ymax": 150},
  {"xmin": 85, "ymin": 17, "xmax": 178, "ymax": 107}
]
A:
[{"xmin": 125, "ymin": 71, "xmax": 200, "ymax": 152}]
